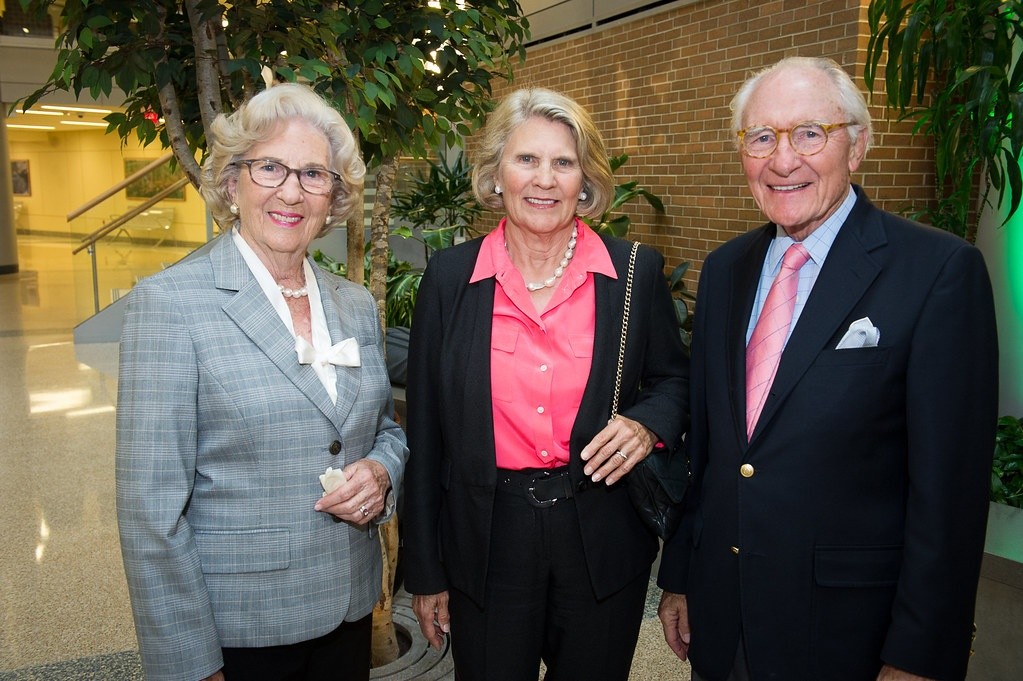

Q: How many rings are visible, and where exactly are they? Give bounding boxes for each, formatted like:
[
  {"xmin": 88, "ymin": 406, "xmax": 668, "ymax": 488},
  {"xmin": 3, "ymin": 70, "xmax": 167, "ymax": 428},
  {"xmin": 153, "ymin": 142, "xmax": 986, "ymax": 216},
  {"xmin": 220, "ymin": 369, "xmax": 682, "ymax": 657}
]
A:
[
  {"xmin": 614, "ymin": 451, "xmax": 629, "ymax": 463},
  {"xmin": 358, "ymin": 505, "xmax": 370, "ymax": 517}
]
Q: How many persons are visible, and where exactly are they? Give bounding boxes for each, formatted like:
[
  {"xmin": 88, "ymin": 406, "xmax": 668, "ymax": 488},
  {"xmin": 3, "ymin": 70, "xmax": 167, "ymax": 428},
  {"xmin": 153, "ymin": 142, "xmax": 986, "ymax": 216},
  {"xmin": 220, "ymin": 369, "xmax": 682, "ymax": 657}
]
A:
[
  {"xmin": 111, "ymin": 80, "xmax": 416, "ymax": 681},
  {"xmin": 394, "ymin": 88, "xmax": 696, "ymax": 680},
  {"xmin": 651, "ymin": 54, "xmax": 1005, "ymax": 681}
]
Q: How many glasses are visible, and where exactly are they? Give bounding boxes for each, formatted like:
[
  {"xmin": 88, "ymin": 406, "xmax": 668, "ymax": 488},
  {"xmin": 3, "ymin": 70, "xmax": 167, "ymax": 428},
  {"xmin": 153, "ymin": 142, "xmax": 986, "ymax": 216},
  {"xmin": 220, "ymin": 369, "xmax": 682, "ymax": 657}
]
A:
[
  {"xmin": 737, "ymin": 120, "xmax": 860, "ymax": 160},
  {"xmin": 229, "ymin": 158, "xmax": 343, "ymax": 195}
]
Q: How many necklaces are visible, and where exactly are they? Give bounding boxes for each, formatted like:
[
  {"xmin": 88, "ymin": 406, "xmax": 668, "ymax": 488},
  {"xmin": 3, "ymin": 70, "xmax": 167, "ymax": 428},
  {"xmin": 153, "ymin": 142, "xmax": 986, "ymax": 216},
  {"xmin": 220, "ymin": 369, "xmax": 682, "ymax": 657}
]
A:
[
  {"xmin": 503, "ymin": 219, "xmax": 578, "ymax": 293},
  {"xmin": 274, "ymin": 282, "xmax": 312, "ymax": 299}
]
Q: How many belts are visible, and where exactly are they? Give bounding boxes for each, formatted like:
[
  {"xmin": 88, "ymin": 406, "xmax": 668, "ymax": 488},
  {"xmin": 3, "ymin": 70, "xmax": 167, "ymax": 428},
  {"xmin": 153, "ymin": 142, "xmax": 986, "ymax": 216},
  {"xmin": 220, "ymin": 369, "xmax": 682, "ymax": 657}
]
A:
[{"xmin": 496, "ymin": 467, "xmax": 574, "ymax": 507}]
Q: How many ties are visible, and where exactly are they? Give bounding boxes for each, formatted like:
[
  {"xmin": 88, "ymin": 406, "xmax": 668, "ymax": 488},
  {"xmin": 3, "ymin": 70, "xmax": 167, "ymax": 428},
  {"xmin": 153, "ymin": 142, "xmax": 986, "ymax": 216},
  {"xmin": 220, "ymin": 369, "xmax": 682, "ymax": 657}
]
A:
[{"xmin": 744, "ymin": 243, "xmax": 811, "ymax": 446}]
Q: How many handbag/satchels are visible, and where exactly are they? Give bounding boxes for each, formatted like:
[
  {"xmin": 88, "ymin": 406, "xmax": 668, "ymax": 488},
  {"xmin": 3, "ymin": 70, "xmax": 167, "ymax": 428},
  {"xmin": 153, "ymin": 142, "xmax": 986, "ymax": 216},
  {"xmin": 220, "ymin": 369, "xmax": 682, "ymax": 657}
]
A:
[{"xmin": 626, "ymin": 436, "xmax": 693, "ymax": 534}]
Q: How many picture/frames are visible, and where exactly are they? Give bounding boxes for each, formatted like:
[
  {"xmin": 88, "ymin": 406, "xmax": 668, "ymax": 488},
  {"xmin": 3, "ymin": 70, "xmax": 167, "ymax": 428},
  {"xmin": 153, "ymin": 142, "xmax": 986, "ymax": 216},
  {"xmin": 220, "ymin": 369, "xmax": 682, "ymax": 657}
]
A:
[
  {"xmin": 124, "ymin": 157, "xmax": 186, "ymax": 201},
  {"xmin": 10, "ymin": 159, "xmax": 31, "ymax": 197}
]
